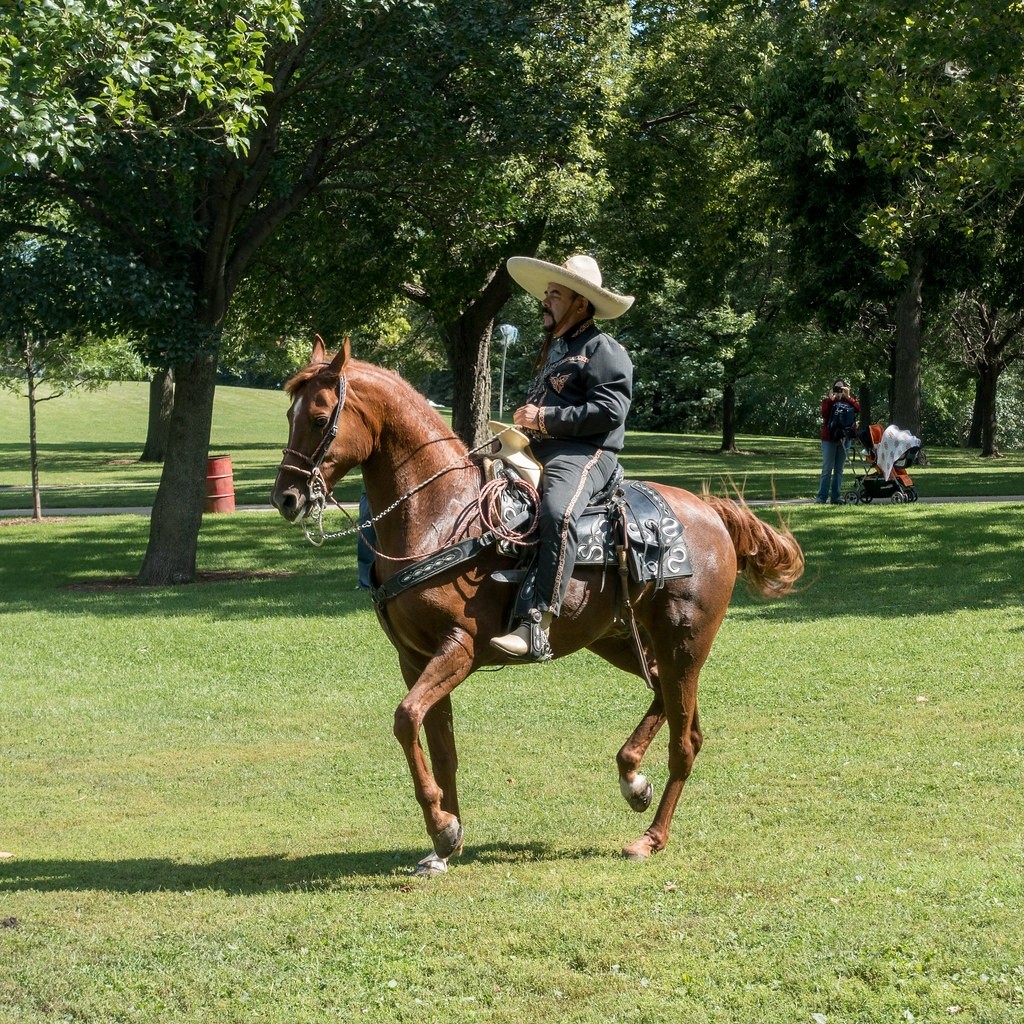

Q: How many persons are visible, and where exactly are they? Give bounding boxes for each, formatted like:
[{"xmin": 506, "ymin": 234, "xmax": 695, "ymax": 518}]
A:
[
  {"xmin": 815, "ymin": 379, "xmax": 861, "ymax": 506},
  {"xmin": 491, "ymin": 255, "xmax": 636, "ymax": 658}
]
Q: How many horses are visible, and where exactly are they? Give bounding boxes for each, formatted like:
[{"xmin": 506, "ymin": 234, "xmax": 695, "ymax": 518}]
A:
[{"xmin": 269, "ymin": 329, "xmax": 822, "ymax": 875}]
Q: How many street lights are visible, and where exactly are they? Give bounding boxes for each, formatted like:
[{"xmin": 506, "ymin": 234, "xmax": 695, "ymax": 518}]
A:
[{"xmin": 497, "ymin": 325, "xmax": 517, "ymax": 419}]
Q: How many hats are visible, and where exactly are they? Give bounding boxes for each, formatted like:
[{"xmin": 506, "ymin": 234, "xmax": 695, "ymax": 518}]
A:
[{"xmin": 506, "ymin": 255, "xmax": 636, "ymax": 320}]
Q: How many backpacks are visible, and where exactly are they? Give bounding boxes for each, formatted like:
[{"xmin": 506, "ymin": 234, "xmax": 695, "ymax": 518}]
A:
[{"xmin": 827, "ymin": 401, "xmax": 856, "ymax": 439}]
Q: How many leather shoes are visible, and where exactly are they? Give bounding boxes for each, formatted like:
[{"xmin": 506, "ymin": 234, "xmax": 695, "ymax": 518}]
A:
[{"xmin": 489, "ymin": 612, "xmax": 553, "ymax": 657}]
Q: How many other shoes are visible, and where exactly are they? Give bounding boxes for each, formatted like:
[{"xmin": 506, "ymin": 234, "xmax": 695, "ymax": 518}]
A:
[{"xmin": 831, "ymin": 500, "xmax": 846, "ymax": 505}]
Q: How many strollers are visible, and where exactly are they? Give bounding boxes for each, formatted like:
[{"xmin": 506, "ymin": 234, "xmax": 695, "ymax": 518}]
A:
[{"xmin": 841, "ymin": 421, "xmax": 927, "ymax": 505}]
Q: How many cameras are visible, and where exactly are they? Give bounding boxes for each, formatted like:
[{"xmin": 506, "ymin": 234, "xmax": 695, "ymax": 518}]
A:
[{"xmin": 833, "ymin": 386, "xmax": 842, "ymax": 393}]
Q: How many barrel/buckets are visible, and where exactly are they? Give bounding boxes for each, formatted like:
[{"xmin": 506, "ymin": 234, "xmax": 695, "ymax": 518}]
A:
[{"xmin": 203, "ymin": 454, "xmax": 236, "ymax": 513}]
